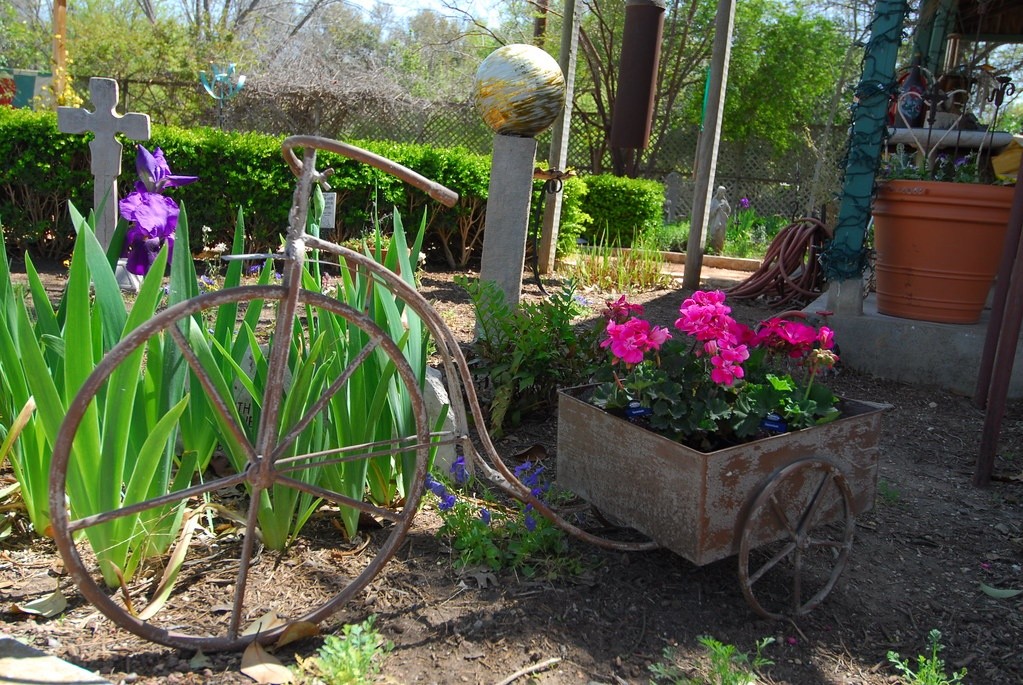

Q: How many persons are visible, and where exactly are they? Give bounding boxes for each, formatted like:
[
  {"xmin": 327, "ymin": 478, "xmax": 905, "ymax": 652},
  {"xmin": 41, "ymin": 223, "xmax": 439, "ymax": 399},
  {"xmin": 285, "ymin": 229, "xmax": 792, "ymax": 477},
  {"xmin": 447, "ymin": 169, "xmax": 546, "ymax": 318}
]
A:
[{"xmin": 709, "ymin": 186, "xmax": 732, "ymax": 255}]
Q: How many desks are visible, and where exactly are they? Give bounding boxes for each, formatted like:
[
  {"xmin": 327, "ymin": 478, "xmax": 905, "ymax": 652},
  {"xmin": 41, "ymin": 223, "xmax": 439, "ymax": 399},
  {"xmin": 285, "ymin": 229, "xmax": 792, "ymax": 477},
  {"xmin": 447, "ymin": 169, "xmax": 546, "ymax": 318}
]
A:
[{"xmin": 847, "ymin": 128, "xmax": 1014, "ymax": 167}]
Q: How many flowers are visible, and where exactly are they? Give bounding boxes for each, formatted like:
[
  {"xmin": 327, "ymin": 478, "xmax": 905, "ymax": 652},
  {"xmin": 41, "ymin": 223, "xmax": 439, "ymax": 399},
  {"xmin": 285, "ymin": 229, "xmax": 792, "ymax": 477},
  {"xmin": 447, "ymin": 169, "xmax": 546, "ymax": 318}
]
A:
[
  {"xmin": 878, "ymin": 153, "xmax": 986, "ymax": 187},
  {"xmin": 590, "ymin": 291, "xmax": 845, "ymax": 441}
]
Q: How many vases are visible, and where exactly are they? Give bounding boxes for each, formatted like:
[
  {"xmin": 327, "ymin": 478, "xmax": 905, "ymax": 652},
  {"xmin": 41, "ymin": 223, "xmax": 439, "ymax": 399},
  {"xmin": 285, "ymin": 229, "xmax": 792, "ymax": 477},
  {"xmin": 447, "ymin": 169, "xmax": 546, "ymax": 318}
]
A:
[{"xmin": 874, "ymin": 180, "xmax": 1016, "ymax": 327}]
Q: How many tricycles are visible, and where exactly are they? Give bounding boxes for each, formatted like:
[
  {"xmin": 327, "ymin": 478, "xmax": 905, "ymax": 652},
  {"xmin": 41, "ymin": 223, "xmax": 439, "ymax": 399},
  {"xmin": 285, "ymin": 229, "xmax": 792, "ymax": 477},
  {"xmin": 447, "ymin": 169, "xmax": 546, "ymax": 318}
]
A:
[{"xmin": 48, "ymin": 135, "xmax": 895, "ymax": 652}]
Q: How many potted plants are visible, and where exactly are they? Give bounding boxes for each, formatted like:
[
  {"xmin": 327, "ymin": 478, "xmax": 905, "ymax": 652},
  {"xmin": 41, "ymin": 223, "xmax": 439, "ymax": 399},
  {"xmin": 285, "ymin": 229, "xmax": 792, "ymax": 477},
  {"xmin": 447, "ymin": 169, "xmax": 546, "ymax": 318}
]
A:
[{"xmin": 339, "ymin": 231, "xmax": 412, "ymax": 298}]
